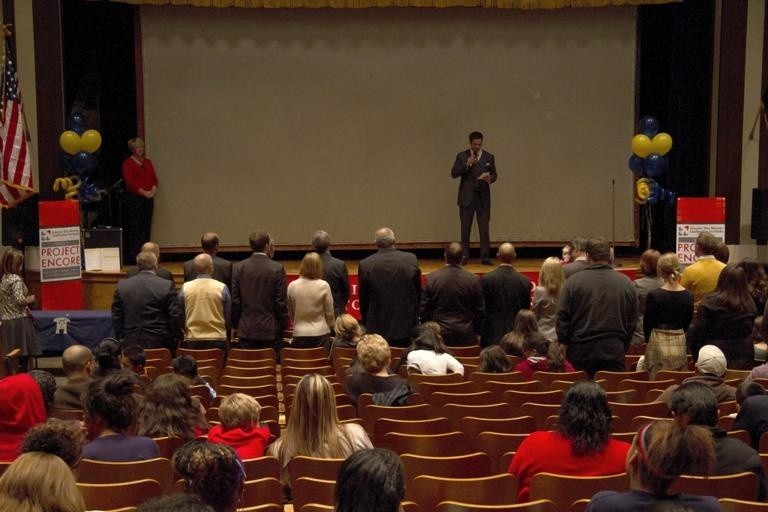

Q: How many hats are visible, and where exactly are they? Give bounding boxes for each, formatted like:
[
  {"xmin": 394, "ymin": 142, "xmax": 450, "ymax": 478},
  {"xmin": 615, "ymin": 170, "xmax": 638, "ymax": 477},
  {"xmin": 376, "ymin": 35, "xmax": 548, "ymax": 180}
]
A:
[{"xmin": 693, "ymin": 344, "xmax": 727, "ymax": 377}]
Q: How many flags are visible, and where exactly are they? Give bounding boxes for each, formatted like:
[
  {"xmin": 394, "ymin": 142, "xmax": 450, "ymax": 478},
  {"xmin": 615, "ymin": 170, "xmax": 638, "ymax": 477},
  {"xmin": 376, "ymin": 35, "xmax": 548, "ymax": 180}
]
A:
[{"xmin": 0, "ymin": 22, "xmax": 34, "ymax": 210}]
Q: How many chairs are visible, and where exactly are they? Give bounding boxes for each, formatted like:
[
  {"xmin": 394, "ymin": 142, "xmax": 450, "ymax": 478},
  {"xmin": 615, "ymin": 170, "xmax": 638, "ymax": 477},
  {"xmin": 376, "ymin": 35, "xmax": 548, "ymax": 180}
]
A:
[
  {"xmin": 208, "ymin": 346, "xmax": 628, "ymax": 511},
  {"xmin": 557, "ymin": 343, "xmax": 768, "ymax": 511},
  {"xmin": 0, "ymin": 345, "xmax": 223, "ymax": 512}
]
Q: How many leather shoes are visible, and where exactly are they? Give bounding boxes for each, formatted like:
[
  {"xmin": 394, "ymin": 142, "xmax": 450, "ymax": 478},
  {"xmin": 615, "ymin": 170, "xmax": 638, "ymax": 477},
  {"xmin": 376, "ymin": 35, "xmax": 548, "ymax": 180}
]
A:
[
  {"xmin": 480, "ymin": 256, "xmax": 491, "ymax": 264},
  {"xmin": 460, "ymin": 256, "xmax": 468, "ymax": 266}
]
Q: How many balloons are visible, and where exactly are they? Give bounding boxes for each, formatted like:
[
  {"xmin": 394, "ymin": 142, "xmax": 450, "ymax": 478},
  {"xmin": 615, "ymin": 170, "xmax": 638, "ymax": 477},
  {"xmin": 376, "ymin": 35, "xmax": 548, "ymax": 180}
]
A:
[
  {"xmin": 53, "ymin": 110, "xmax": 103, "ymax": 204},
  {"xmin": 627, "ymin": 116, "xmax": 676, "ymax": 208}
]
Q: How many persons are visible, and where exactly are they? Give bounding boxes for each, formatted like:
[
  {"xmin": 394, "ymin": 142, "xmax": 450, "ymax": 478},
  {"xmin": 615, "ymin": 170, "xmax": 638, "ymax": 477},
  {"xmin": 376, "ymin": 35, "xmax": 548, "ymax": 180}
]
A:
[
  {"xmin": 531, "ymin": 256, "xmax": 567, "ymax": 344},
  {"xmin": 125, "ymin": 242, "xmax": 176, "ymax": 282},
  {"xmin": 500, "ymin": 309, "xmax": 549, "ymax": 356},
  {"xmin": 122, "ymin": 138, "xmax": 158, "ymax": 267},
  {"xmin": 556, "ymin": 237, "xmax": 640, "ymax": 378},
  {"xmin": 268, "ymin": 237, "xmax": 275, "ymax": 258},
  {"xmin": 451, "ymin": 131, "xmax": 497, "ymax": 267},
  {"xmin": 345, "ymin": 334, "xmax": 412, "ymax": 417},
  {"xmin": 508, "ymin": 379, "xmax": 632, "ymax": 503},
  {"xmin": 422, "ymin": 242, "xmax": 484, "ymax": 347},
  {"xmin": 329, "ymin": 313, "xmax": 368, "ymax": 360},
  {"xmin": 631, "ymin": 247, "xmax": 666, "ymax": 347},
  {"xmin": 332, "ymin": 448, "xmax": 406, "ymax": 512},
  {"xmin": 231, "ymin": 230, "xmax": 288, "ymax": 362},
  {"xmin": 181, "ymin": 231, "xmax": 232, "ymax": 289},
  {"xmin": 729, "ymin": 381, "xmax": 767, "ymax": 416},
  {"xmin": 406, "ymin": 324, "xmax": 463, "ymax": 376},
  {"xmin": 358, "ymin": 227, "xmax": 422, "ymax": 349},
  {"xmin": 119, "ymin": 345, "xmax": 148, "ymax": 374},
  {"xmin": 561, "ymin": 237, "xmax": 595, "ymax": 278},
  {"xmin": 586, "ymin": 413, "xmax": 720, "ymax": 512},
  {"xmin": 1, "ymin": 374, "xmax": 46, "ymax": 466},
  {"xmin": 478, "ymin": 346, "xmax": 512, "ymax": 374},
  {"xmin": 53, "ymin": 345, "xmax": 99, "ymax": 411},
  {"xmin": 737, "ymin": 258, "xmax": 767, "ymax": 317},
  {"xmin": 680, "ymin": 231, "xmax": 729, "ymax": 326},
  {"xmin": 674, "ymin": 345, "xmax": 738, "ymax": 406},
  {"xmin": 111, "ymin": 251, "xmax": 182, "ymax": 360},
  {"xmin": 715, "ymin": 242, "xmax": 730, "ymax": 264},
  {"xmin": 642, "ymin": 253, "xmax": 694, "ymax": 380},
  {"xmin": 22, "ymin": 418, "xmax": 88, "ymax": 483},
  {"xmin": 731, "ymin": 394, "xmax": 767, "ymax": 452},
  {"xmin": 172, "ymin": 354, "xmax": 217, "ymax": 398},
  {"xmin": 138, "ymin": 373, "xmax": 208, "ymax": 439},
  {"xmin": 309, "ymin": 230, "xmax": 350, "ymax": 336},
  {"xmin": 479, "ymin": 242, "xmax": 532, "ymax": 349},
  {"xmin": 0, "ymin": 246, "xmax": 41, "ymax": 361},
  {"xmin": 91, "ymin": 338, "xmax": 141, "ymax": 387},
  {"xmin": 29, "ymin": 369, "xmax": 56, "ymax": 416},
  {"xmin": 0, "ymin": 452, "xmax": 85, "ymax": 511},
  {"xmin": 178, "ymin": 252, "xmax": 232, "ymax": 352},
  {"xmin": 207, "ymin": 393, "xmax": 270, "ymax": 460},
  {"xmin": 82, "ymin": 370, "xmax": 160, "ymax": 463},
  {"xmin": 686, "ymin": 264, "xmax": 757, "ymax": 370},
  {"xmin": 665, "ymin": 380, "xmax": 766, "ymax": 501},
  {"xmin": 171, "ymin": 437, "xmax": 248, "ymax": 512},
  {"xmin": 266, "ymin": 373, "xmax": 373, "ymax": 499},
  {"xmin": 393, "ymin": 321, "xmax": 455, "ymax": 378},
  {"xmin": 745, "ymin": 363, "xmax": 768, "ymax": 383},
  {"xmin": 286, "ymin": 252, "xmax": 336, "ymax": 350},
  {"xmin": 514, "ymin": 332, "xmax": 575, "ymax": 382}
]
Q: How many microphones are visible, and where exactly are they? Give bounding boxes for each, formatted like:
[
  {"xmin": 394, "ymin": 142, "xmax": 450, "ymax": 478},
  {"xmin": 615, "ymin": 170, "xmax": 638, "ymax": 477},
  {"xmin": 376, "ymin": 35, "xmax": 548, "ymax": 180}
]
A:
[{"xmin": 472, "ymin": 149, "xmax": 478, "ymax": 167}]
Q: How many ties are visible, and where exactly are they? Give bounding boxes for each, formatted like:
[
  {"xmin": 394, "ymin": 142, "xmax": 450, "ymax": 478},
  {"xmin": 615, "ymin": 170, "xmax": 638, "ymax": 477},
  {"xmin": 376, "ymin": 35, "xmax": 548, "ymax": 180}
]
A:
[{"xmin": 473, "ymin": 152, "xmax": 477, "ymax": 163}]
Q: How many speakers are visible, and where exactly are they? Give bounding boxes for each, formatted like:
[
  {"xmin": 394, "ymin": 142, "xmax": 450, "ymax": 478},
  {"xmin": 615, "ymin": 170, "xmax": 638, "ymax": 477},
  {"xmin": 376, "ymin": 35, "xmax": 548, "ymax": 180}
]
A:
[{"xmin": 82, "ymin": 225, "xmax": 124, "ymax": 270}]
